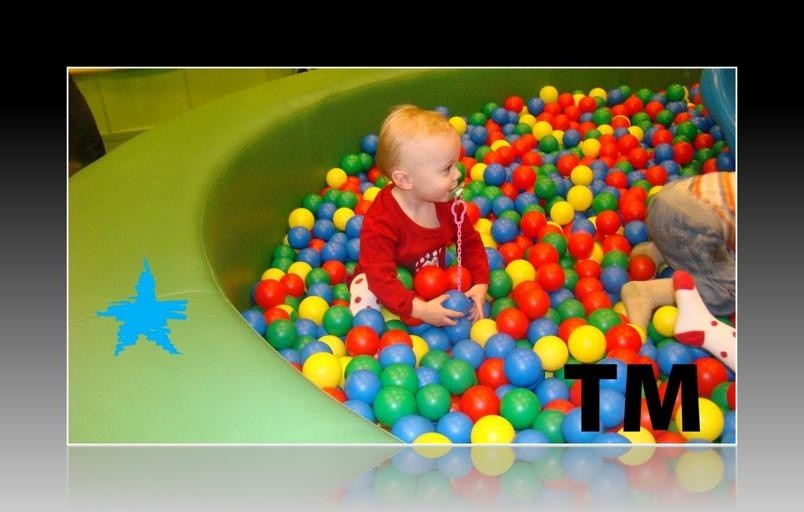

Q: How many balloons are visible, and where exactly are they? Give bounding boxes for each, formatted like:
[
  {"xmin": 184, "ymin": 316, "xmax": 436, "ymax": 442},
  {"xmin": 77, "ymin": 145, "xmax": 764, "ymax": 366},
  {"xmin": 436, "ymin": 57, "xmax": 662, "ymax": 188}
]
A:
[{"xmin": 242, "ymin": 83, "xmax": 736, "ymax": 445}]
